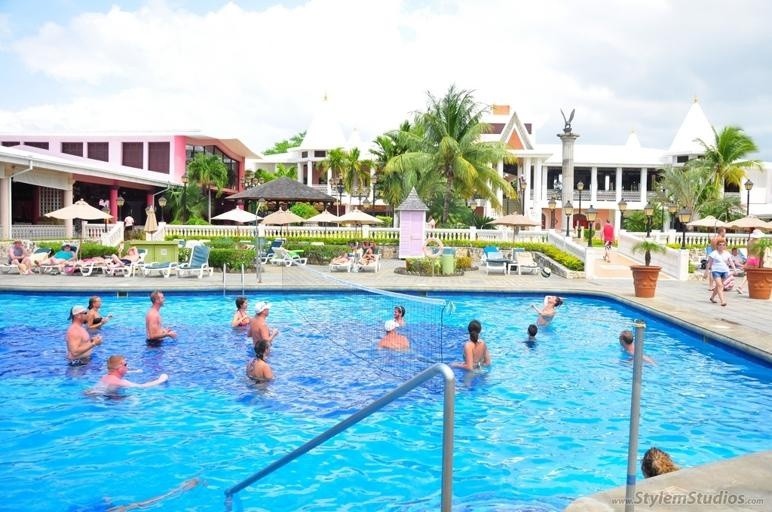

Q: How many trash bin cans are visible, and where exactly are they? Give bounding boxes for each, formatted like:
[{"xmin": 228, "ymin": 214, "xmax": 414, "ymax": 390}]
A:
[{"xmin": 442, "ymin": 254, "xmax": 454, "ymax": 275}]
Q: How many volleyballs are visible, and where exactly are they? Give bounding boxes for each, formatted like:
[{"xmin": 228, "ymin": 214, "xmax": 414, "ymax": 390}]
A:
[{"xmin": 541, "ymin": 267, "xmax": 551, "ymax": 277}]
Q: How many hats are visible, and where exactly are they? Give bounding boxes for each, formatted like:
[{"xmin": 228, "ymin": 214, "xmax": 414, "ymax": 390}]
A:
[
  {"xmin": 254, "ymin": 301, "xmax": 272, "ymax": 314},
  {"xmin": 384, "ymin": 320, "xmax": 399, "ymax": 331},
  {"xmin": 749, "ymin": 229, "xmax": 765, "ymax": 238},
  {"xmin": 68, "ymin": 305, "xmax": 87, "ymax": 320}
]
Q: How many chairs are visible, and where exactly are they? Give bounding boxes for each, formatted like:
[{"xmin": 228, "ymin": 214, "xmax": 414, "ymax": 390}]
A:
[
  {"xmin": 480, "ymin": 245, "xmax": 507, "ymax": 275},
  {"xmin": 512, "ymin": 246, "xmax": 540, "ymax": 276},
  {"xmin": 1, "ymin": 237, "xmax": 384, "ymax": 279}
]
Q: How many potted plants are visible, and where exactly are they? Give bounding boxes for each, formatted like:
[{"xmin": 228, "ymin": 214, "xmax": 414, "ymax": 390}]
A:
[
  {"xmin": 628, "ymin": 238, "xmax": 666, "ymax": 298},
  {"xmin": 742, "ymin": 236, "xmax": 772, "ymax": 299}
]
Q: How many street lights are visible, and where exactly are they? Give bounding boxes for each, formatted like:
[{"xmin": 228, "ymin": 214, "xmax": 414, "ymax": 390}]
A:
[
  {"xmin": 254, "ymin": 195, "xmax": 266, "ymax": 282},
  {"xmin": 519, "ymin": 173, "xmax": 527, "ymax": 230},
  {"xmin": 240, "ymin": 175, "xmax": 252, "ymax": 225},
  {"xmin": 158, "ymin": 194, "xmax": 167, "ymax": 222},
  {"xmin": 645, "ymin": 200, "xmax": 654, "ymax": 236},
  {"xmin": 337, "ymin": 178, "xmax": 345, "ymax": 227},
  {"xmin": 371, "ymin": 172, "xmax": 377, "ymax": 217},
  {"xmin": 585, "ymin": 204, "xmax": 598, "ymax": 247},
  {"xmin": 182, "ymin": 171, "xmax": 190, "ymax": 223},
  {"xmin": 618, "ymin": 197, "xmax": 627, "ymax": 229},
  {"xmin": 503, "ymin": 173, "xmax": 511, "ymax": 214},
  {"xmin": 744, "ymin": 178, "xmax": 753, "ymax": 233},
  {"xmin": 675, "ymin": 205, "xmax": 692, "ymax": 250},
  {"xmin": 253, "ymin": 177, "xmax": 264, "ymax": 216},
  {"xmin": 564, "ymin": 201, "xmax": 573, "ymax": 236},
  {"xmin": 576, "ymin": 178, "xmax": 585, "ymax": 237}
]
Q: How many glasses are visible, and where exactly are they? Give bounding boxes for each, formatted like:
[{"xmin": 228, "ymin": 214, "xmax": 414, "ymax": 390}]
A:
[{"xmin": 80, "ymin": 312, "xmax": 87, "ymax": 314}]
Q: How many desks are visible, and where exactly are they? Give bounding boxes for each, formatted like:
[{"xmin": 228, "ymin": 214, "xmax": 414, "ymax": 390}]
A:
[{"xmin": 508, "ymin": 260, "xmax": 521, "ymax": 275}]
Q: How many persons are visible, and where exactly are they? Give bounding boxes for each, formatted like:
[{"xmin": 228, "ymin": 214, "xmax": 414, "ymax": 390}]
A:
[
  {"xmin": 703, "ymin": 227, "xmax": 766, "ymax": 307},
  {"xmin": 67, "ymin": 477, "xmax": 200, "ymax": 512},
  {"xmin": 641, "ymin": 448, "xmax": 681, "ymax": 479},
  {"xmin": 619, "ymin": 330, "xmax": 656, "ymax": 366},
  {"xmin": 247, "ymin": 339, "xmax": 273, "ymax": 387},
  {"xmin": 378, "ymin": 319, "xmax": 410, "ymax": 352},
  {"xmin": 427, "ymin": 215, "xmax": 436, "ymax": 229},
  {"xmin": 6, "ymin": 239, "xmax": 140, "ymax": 274},
  {"xmin": 330, "ymin": 240, "xmax": 380, "ymax": 265},
  {"xmin": 124, "ymin": 212, "xmax": 135, "ymax": 240},
  {"xmin": 600, "ymin": 219, "xmax": 614, "ymax": 262},
  {"xmin": 247, "ymin": 302, "xmax": 279, "ymax": 347},
  {"xmin": 145, "ymin": 289, "xmax": 178, "ymax": 347},
  {"xmin": 232, "ymin": 297, "xmax": 250, "ymax": 327},
  {"xmin": 87, "ymin": 296, "xmax": 112, "ymax": 330},
  {"xmin": 82, "ymin": 356, "xmax": 168, "ymax": 400},
  {"xmin": 393, "ymin": 306, "xmax": 405, "ymax": 326},
  {"xmin": 450, "ymin": 320, "xmax": 490, "ymax": 388},
  {"xmin": 530, "ymin": 295, "xmax": 568, "ymax": 327},
  {"xmin": 66, "ymin": 305, "xmax": 103, "ymax": 368},
  {"xmin": 522, "ymin": 325, "xmax": 540, "ymax": 350}
]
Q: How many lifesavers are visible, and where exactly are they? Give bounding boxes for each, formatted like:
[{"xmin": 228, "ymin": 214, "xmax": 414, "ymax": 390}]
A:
[
  {"xmin": 63, "ymin": 258, "xmax": 76, "ymax": 274},
  {"xmin": 80, "ymin": 258, "xmax": 95, "ymax": 264},
  {"xmin": 423, "ymin": 238, "xmax": 443, "ymax": 258}
]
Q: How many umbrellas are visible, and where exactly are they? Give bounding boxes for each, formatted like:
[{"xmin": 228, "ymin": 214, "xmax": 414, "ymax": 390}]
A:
[
  {"xmin": 485, "ymin": 211, "xmax": 544, "ymax": 249},
  {"xmin": 43, "ymin": 198, "xmax": 114, "ymax": 259},
  {"xmin": 210, "ymin": 206, "xmax": 384, "ymax": 226},
  {"xmin": 685, "ymin": 214, "xmax": 772, "ymax": 232},
  {"xmin": 144, "ymin": 206, "xmax": 158, "ymax": 240}
]
